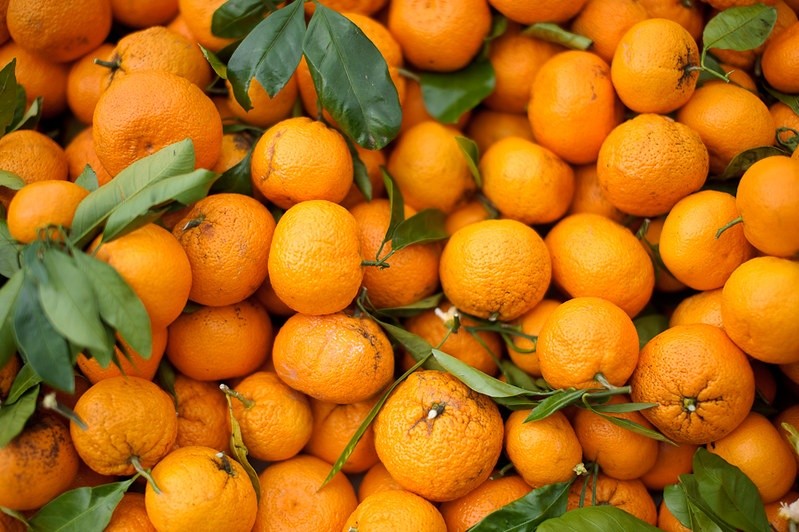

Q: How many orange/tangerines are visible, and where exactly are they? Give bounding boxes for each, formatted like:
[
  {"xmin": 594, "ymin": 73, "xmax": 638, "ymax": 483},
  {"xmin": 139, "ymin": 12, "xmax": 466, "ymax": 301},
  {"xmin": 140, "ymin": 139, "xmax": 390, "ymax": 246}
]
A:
[{"xmin": 0, "ymin": 0, "xmax": 799, "ymax": 532}]
